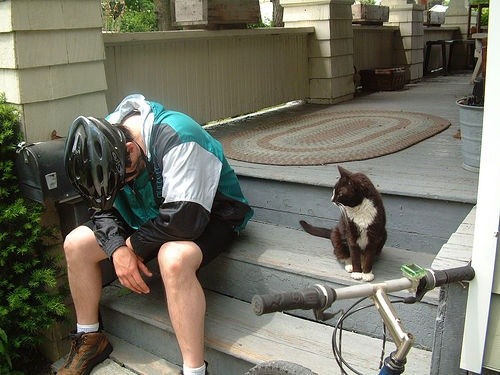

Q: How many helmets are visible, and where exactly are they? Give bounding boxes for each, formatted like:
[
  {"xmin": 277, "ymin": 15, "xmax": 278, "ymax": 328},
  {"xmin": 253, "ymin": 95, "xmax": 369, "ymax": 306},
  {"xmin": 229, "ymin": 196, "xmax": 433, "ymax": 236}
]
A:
[{"xmin": 65, "ymin": 115, "xmax": 126, "ymax": 209}]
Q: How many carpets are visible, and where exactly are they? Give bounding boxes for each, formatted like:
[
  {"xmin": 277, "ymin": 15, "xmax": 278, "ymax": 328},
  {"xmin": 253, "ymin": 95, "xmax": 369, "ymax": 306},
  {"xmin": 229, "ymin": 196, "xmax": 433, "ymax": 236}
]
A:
[{"xmin": 215, "ymin": 110, "xmax": 452, "ymax": 167}]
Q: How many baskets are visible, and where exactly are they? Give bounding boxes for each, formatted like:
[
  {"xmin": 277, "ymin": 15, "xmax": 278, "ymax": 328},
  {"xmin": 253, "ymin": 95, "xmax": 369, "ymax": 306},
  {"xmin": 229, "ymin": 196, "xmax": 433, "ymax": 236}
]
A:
[{"xmin": 370, "ymin": 66, "xmax": 406, "ymax": 91}]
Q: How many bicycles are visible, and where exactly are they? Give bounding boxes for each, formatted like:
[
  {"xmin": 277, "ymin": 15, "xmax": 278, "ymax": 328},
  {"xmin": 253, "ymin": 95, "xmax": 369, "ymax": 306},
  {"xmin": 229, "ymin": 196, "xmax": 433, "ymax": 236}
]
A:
[{"xmin": 246, "ymin": 264, "xmax": 475, "ymax": 374}]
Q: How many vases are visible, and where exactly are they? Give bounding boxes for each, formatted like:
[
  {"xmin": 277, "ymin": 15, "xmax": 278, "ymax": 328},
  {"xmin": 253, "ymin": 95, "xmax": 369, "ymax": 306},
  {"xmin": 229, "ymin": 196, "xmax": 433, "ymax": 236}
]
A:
[{"xmin": 455, "ymin": 95, "xmax": 484, "ymax": 174}]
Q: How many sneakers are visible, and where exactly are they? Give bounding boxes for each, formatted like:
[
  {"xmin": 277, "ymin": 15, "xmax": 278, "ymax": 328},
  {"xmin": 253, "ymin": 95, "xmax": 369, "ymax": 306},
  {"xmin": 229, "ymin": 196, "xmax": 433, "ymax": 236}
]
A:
[{"xmin": 56, "ymin": 331, "xmax": 113, "ymax": 375}]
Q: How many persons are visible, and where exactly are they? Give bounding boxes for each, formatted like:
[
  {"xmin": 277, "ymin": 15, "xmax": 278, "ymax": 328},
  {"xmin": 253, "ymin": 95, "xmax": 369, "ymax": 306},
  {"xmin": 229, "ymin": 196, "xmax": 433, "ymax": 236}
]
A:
[{"xmin": 57, "ymin": 94, "xmax": 255, "ymax": 371}]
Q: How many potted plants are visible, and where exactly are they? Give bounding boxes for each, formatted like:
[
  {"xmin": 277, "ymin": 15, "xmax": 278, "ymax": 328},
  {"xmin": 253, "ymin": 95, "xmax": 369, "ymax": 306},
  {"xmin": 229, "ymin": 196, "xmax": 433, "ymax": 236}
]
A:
[{"xmin": 351, "ymin": 0, "xmax": 389, "ymax": 24}]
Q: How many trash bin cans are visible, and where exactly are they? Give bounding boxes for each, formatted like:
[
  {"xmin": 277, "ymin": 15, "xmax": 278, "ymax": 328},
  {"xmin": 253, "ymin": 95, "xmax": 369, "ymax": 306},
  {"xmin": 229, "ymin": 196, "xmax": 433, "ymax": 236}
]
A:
[{"xmin": 14, "ymin": 137, "xmax": 118, "ymax": 292}]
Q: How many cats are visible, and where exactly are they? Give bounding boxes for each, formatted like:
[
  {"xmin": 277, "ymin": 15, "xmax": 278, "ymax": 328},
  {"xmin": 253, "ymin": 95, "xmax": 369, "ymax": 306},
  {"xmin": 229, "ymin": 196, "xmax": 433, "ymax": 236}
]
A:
[{"xmin": 299, "ymin": 163, "xmax": 388, "ymax": 283}]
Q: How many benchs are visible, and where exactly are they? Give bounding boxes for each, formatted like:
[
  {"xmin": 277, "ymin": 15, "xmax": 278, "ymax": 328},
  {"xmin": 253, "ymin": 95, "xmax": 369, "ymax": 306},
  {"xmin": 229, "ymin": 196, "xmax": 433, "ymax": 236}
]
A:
[{"xmin": 423, "ymin": 38, "xmax": 476, "ymax": 76}]
